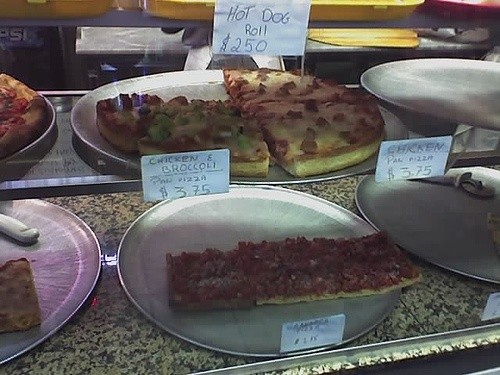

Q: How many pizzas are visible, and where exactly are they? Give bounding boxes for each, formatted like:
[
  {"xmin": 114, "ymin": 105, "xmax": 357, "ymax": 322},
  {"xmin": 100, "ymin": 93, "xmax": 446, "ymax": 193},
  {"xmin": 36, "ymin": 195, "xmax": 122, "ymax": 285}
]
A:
[
  {"xmin": 1, "ymin": 72, "xmax": 50, "ymax": 154},
  {"xmin": 165, "ymin": 230, "xmax": 424, "ymax": 312},
  {"xmin": 1, "ymin": 259, "xmax": 42, "ymax": 331},
  {"xmin": 95, "ymin": 65, "xmax": 384, "ymax": 176}
]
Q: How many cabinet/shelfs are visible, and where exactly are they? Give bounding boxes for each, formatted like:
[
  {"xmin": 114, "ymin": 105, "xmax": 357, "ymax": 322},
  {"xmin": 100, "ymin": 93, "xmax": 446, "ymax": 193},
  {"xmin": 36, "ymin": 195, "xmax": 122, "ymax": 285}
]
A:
[{"xmin": 0, "ymin": 8, "xmax": 500, "ymax": 375}]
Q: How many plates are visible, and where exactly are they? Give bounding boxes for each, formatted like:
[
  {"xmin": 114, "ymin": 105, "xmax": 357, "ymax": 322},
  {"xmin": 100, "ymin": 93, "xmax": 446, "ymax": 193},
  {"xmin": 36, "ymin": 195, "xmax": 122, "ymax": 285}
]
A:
[
  {"xmin": 352, "ymin": 163, "xmax": 500, "ymax": 283},
  {"xmin": 115, "ymin": 181, "xmax": 403, "ymax": 358},
  {"xmin": 1, "ymin": 91, "xmax": 57, "ymax": 163},
  {"xmin": 69, "ymin": 67, "xmax": 410, "ymax": 186},
  {"xmin": 357, "ymin": 56, "xmax": 500, "ymax": 134},
  {"xmin": 0, "ymin": 198, "xmax": 103, "ymax": 364}
]
